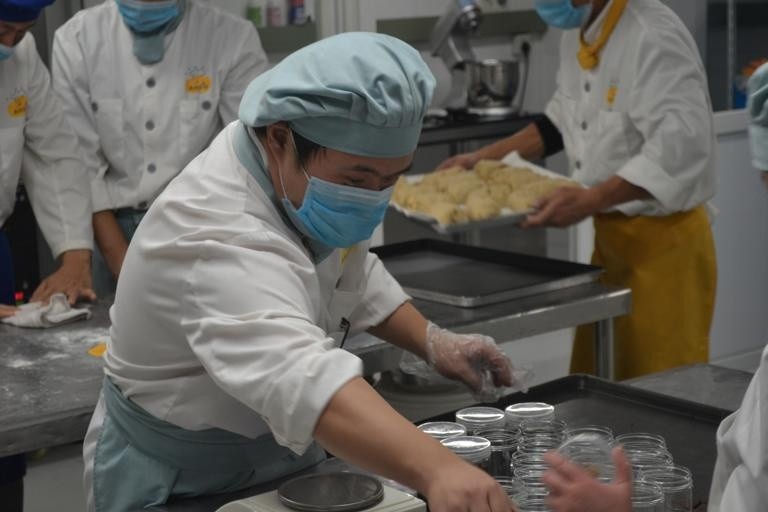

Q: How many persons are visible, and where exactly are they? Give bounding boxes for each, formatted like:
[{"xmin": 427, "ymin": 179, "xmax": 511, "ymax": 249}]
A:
[
  {"xmin": 0, "ymin": 0, "xmax": 97, "ymax": 512},
  {"xmin": 50, "ymin": 0, "xmax": 275, "ymax": 290},
  {"xmin": 83, "ymin": 31, "xmax": 522, "ymax": 512},
  {"xmin": 434, "ymin": 1, "xmax": 720, "ymax": 386},
  {"xmin": 537, "ymin": 55, "xmax": 768, "ymax": 512}
]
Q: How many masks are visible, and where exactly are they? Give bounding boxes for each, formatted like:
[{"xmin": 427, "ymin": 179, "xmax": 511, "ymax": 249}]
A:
[
  {"xmin": 535, "ymin": 0, "xmax": 594, "ymax": 30},
  {"xmin": 1, "ymin": 42, "xmax": 16, "ymax": 61},
  {"xmin": 115, "ymin": 1, "xmax": 179, "ymax": 33},
  {"xmin": 279, "ymin": 164, "xmax": 395, "ymax": 249}
]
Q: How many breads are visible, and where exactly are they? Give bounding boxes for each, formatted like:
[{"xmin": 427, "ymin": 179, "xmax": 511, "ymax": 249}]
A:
[{"xmin": 389, "ymin": 159, "xmax": 578, "ymax": 224}]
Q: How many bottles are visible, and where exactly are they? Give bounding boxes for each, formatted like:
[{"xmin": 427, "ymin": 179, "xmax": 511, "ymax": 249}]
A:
[
  {"xmin": 415, "ymin": 401, "xmax": 695, "ymax": 511},
  {"xmin": 247, "ymin": 1, "xmax": 305, "ymax": 28}
]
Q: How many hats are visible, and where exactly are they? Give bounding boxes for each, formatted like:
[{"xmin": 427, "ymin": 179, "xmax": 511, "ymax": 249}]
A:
[
  {"xmin": 746, "ymin": 62, "xmax": 768, "ymax": 172},
  {"xmin": 0, "ymin": 0, "xmax": 54, "ymax": 21},
  {"xmin": 238, "ymin": 31, "xmax": 437, "ymax": 158}
]
